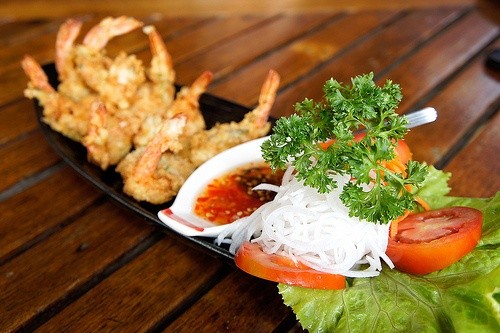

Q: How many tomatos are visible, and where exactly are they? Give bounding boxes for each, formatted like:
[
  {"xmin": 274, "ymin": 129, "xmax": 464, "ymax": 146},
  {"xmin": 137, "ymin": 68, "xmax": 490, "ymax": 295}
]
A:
[{"xmin": 231, "ymin": 130, "xmax": 482, "ymax": 291}]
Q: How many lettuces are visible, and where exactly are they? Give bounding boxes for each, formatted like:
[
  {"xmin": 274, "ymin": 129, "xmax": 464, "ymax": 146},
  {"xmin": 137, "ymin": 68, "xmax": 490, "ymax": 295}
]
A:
[{"xmin": 274, "ymin": 162, "xmax": 500, "ymax": 333}]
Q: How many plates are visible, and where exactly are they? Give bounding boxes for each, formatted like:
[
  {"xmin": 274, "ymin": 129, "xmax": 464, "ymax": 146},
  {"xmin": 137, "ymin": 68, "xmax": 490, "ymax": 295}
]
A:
[{"xmin": 27, "ymin": 57, "xmax": 287, "ymax": 263}]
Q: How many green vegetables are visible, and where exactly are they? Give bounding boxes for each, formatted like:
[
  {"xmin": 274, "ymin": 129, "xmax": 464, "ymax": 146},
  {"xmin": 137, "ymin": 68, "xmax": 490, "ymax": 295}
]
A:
[{"xmin": 260, "ymin": 73, "xmax": 423, "ymax": 220}]
